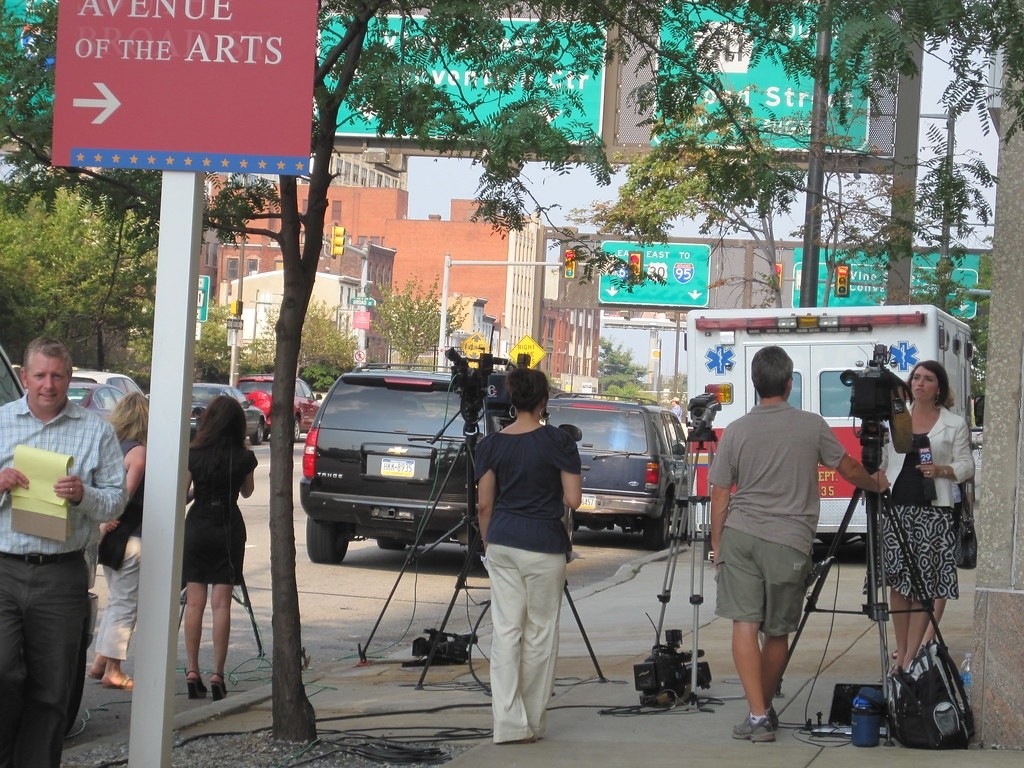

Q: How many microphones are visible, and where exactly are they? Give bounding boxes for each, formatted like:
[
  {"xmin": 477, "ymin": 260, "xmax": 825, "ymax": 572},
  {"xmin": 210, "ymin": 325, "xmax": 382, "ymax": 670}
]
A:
[{"xmin": 491, "ymin": 358, "xmax": 509, "ymax": 366}]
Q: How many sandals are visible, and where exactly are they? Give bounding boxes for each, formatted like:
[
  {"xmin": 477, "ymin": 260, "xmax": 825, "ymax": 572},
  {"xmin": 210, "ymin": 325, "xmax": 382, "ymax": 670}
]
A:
[{"xmin": 877, "ymin": 664, "xmax": 901, "ymax": 681}]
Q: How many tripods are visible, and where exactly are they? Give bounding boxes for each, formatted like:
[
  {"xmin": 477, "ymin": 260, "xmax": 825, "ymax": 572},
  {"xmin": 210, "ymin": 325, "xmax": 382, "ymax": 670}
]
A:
[
  {"xmin": 774, "ymin": 420, "xmax": 975, "ymax": 748},
  {"xmin": 357, "ymin": 392, "xmax": 608, "ymax": 691},
  {"xmin": 646, "ymin": 392, "xmax": 723, "ymax": 711}
]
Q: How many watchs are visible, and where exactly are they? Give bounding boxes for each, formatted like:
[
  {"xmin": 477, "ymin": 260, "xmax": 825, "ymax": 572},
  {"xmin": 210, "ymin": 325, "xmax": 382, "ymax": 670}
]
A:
[{"xmin": 942, "ymin": 466, "xmax": 948, "ymax": 478}]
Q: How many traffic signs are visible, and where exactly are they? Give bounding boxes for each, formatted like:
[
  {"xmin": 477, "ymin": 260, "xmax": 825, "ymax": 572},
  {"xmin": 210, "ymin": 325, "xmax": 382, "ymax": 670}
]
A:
[{"xmin": 226, "ymin": 319, "xmax": 245, "ymax": 329}]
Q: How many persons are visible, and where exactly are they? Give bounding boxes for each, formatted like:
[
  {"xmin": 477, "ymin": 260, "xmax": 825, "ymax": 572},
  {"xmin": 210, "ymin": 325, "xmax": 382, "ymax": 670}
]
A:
[
  {"xmin": 706, "ymin": 346, "xmax": 891, "ymax": 743},
  {"xmin": 182, "ymin": 396, "xmax": 258, "ymax": 702},
  {"xmin": 0, "ymin": 338, "xmax": 129, "ymax": 768},
  {"xmin": 89, "ymin": 391, "xmax": 149, "ymax": 690},
  {"xmin": 669, "ymin": 397, "xmax": 683, "ymax": 424},
  {"xmin": 875, "ymin": 360, "xmax": 975, "ymax": 679},
  {"xmin": 473, "ymin": 369, "xmax": 582, "ymax": 745}
]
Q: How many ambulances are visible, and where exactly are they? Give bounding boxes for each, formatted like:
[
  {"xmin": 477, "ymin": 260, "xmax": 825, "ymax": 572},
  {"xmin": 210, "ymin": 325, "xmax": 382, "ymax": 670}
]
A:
[{"xmin": 686, "ymin": 305, "xmax": 986, "ymax": 570}]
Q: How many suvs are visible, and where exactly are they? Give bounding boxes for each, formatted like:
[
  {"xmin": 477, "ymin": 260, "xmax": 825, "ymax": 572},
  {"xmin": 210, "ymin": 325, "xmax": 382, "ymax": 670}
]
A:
[
  {"xmin": 300, "ymin": 361, "xmax": 583, "ymax": 564},
  {"xmin": 546, "ymin": 392, "xmax": 688, "ymax": 550}
]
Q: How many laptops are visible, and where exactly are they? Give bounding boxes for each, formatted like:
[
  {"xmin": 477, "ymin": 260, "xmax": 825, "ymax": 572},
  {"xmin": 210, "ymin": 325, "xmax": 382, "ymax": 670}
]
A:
[{"xmin": 809, "ymin": 683, "xmax": 888, "ymax": 738}]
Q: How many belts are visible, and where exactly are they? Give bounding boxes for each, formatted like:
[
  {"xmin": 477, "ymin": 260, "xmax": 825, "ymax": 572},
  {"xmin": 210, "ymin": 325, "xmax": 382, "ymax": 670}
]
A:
[{"xmin": 0, "ymin": 549, "xmax": 85, "ymax": 566}]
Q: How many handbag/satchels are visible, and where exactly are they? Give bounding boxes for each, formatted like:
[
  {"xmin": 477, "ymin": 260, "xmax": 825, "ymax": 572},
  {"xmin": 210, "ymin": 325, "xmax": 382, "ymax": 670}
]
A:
[{"xmin": 950, "ymin": 481, "xmax": 976, "ymax": 571}]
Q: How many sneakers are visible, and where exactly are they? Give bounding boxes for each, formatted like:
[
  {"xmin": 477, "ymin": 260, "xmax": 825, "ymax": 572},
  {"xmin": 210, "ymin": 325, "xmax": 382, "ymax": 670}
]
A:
[
  {"xmin": 732, "ymin": 712, "xmax": 776, "ymax": 743},
  {"xmin": 764, "ymin": 708, "xmax": 780, "ymax": 730}
]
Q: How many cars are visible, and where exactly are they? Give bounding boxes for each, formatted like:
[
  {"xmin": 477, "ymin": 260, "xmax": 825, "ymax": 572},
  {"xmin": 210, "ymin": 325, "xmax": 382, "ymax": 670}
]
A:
[
  {"xmin": 70, "ymin": 372, "xmax": 147, "ymax": 396},
  {"xmin": 191, "ymin": 383, "xmax": 267, "ymax": 445},
  {"xmin": 235, "ymin": 376, "xmax": 324, "ymax": 441},
  {"xmin": 66, "ymin": 382, "xmax": 127, "ymax": 419},
  {"xmin": 0, "ymin": 343, "xmax": 25, "ymax": 406}
]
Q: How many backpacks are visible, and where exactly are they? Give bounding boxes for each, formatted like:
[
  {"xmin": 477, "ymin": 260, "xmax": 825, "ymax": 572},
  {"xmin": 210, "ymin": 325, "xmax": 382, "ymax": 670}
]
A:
[{"xmin": 886, "ymin": 637, "xmax": 973, "ymax": 750}]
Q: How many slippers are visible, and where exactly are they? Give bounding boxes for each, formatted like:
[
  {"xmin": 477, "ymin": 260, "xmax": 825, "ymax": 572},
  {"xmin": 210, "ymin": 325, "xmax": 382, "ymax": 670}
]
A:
[
  {"xmin": 101, "ymin": 674, "xmax": 133, "ymax": 690},
  {"xmin": 87, "ymin": 664, "xmax": 107, "ymax": 680}
]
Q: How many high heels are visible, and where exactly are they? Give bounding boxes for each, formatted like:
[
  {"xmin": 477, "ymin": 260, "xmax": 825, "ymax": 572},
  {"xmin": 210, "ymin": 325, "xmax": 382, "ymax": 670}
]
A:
[
  {"xmin": 210, "ymin": 673, "xmax": 227, "ymax": 701},
  {"xmin": 185, "ymin": 670, "xmax": 208, "ymax": 700}
]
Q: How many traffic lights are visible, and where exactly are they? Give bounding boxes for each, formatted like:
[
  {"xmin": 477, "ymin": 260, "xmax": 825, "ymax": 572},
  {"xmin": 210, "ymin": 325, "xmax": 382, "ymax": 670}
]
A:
[
  {"xmin": 335, "ymin": 226, "xmax": 346, "ymax": 255},
  {"xmin": 563, "ymin": 249, "xmax": 576, "ymax": 279},
  {"xmin": 835, "ymin": 263, "xmax": 850, "ymax": 296},
  {"xmin": 628, "ymin": 251, "xmax": 644, "ymax": 285},
  {"xmin": 775, "ymin": 262, "xmax": 783, "ymax": 288}
]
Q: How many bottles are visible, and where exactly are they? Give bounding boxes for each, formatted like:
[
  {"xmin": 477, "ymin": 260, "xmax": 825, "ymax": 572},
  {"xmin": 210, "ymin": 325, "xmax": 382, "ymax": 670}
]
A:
[
  {"xmin": 853, "ymin": 697, "xmax": 871, "ymax": 708},
  {"xmin": 958, "ymin": 654, "xmax": 973, "ymax": 707}
]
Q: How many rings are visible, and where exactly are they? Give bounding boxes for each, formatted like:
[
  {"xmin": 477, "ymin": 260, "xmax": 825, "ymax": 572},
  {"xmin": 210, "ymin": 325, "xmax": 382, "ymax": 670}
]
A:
[
  {"xmin": 927, "ymin": 471, "xmax": 930, "ymax": 474},
  {"xmin": 70, "ymin": 487, "xmax": 73, "ymax": 494}
]
